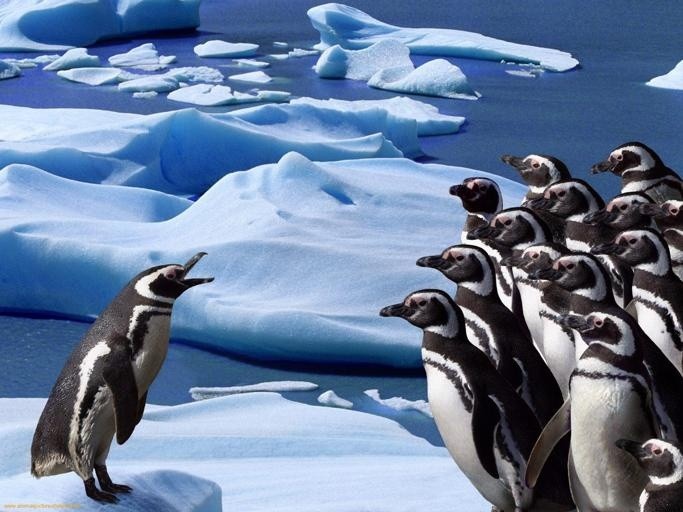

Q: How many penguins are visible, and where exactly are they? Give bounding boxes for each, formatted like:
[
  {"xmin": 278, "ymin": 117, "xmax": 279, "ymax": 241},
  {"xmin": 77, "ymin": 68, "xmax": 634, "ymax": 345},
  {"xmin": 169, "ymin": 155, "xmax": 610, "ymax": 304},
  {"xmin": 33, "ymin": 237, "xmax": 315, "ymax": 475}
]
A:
[
  {"xmin": 380, "ymin": 140, "xmax": 683, "ymax": 512},
  {"xmin": 30, "ymin": 249, "xmax": 216, "ymax": 506}
]
